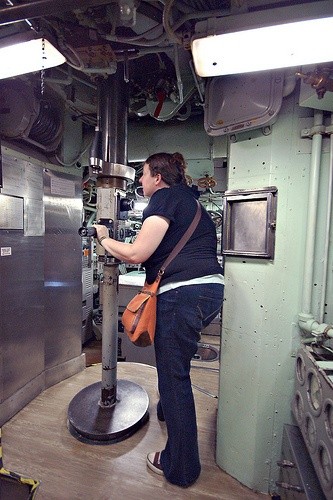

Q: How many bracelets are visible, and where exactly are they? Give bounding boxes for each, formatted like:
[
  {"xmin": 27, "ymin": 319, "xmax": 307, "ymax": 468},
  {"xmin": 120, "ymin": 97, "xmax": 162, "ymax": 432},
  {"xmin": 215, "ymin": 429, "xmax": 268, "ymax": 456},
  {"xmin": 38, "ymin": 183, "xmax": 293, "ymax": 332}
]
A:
[{"xmin": 97, "ymin": 234, "xmax": 107, "ymax": 247}]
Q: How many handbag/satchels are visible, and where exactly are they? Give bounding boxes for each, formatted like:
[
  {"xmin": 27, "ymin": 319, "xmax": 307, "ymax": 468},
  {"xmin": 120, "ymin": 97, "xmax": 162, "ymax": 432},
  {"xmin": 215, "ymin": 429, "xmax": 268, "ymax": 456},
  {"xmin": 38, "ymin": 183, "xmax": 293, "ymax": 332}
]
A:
[{"xmin": 122, "ymin": 275, "xmax": 161, "ymax": 348}]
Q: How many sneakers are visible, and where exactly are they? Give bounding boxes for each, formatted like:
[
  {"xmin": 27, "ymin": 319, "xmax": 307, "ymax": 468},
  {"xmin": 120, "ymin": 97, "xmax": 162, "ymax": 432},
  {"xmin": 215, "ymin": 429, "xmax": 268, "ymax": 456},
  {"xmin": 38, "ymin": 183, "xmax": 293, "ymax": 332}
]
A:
[{"xmin": 147, "ymin": 452, "xmax": 166, "ymax": 476}]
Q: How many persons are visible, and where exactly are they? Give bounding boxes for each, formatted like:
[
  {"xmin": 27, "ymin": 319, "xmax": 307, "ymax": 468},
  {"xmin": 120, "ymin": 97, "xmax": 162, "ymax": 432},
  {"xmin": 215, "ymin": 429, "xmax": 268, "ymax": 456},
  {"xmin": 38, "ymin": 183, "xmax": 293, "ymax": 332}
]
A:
[{"xmin": 86, "ymin": 150, "xmax": 228, "ymax": 489}]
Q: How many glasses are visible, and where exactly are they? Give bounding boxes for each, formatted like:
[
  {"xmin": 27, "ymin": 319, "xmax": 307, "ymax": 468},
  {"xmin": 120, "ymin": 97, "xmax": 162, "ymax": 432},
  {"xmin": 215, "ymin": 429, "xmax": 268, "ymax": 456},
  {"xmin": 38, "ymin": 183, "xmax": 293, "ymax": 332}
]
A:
[{"xmin": 150, "ymin": 166, "xmax": 184, "ymax": 184}]
